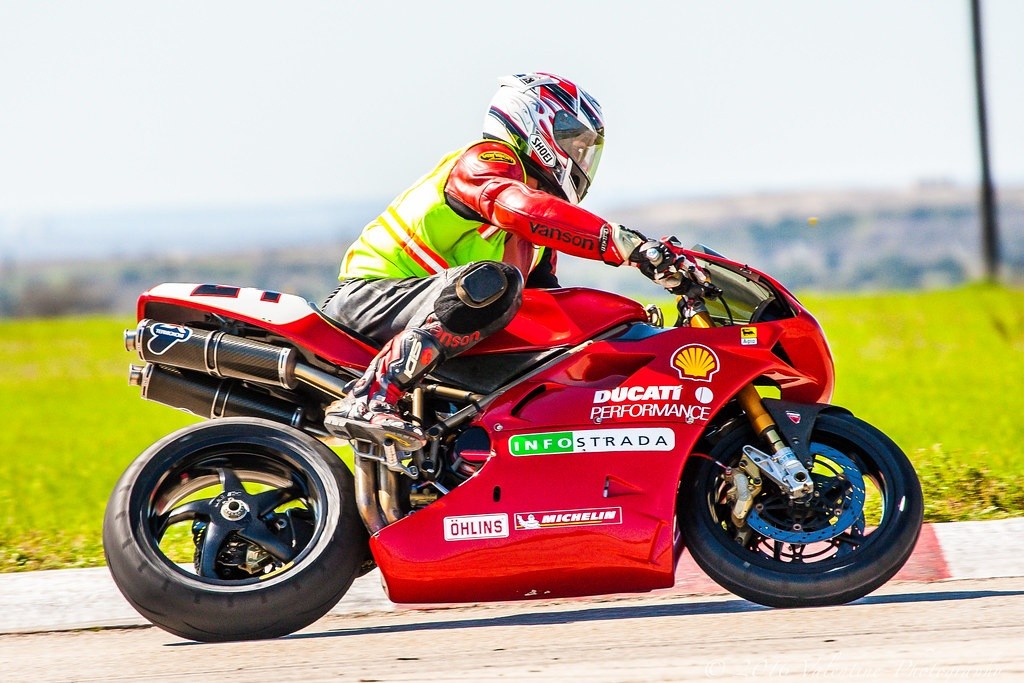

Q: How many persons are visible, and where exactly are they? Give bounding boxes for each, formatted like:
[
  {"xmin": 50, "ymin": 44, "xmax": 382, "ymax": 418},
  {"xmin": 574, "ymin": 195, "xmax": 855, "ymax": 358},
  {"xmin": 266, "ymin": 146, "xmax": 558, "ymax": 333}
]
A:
[{"xmin": 321, "ymin": 71, "xmax": 706, "ymax": 451}]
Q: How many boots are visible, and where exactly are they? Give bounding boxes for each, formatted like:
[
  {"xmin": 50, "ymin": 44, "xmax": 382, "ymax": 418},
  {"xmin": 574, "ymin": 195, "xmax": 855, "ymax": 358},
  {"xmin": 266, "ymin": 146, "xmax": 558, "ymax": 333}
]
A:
[{"xmin": 324, "ymin": 327, "xmax": 446, "ymax": 452}]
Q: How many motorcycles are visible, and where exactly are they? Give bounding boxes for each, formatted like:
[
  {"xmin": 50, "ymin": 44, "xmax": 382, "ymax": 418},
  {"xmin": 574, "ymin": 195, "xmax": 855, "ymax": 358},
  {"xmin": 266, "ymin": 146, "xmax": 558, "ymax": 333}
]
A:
[{"xmin": 101, "ymin": 236, "xmax": 926, "ymax": 642}]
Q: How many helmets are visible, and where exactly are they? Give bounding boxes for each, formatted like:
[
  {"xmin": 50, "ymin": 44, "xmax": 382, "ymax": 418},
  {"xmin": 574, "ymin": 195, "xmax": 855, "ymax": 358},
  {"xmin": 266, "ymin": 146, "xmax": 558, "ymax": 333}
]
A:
[{"xmin": 483, "ymin": 71, "xmax": 605, "ymax": 204}]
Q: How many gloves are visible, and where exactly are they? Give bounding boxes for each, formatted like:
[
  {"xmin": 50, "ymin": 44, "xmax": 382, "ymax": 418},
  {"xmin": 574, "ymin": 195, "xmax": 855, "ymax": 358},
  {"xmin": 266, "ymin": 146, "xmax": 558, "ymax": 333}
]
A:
[{"xmin": 614, "ymin": 224, "xmax": 722, "ymax": 301}]
